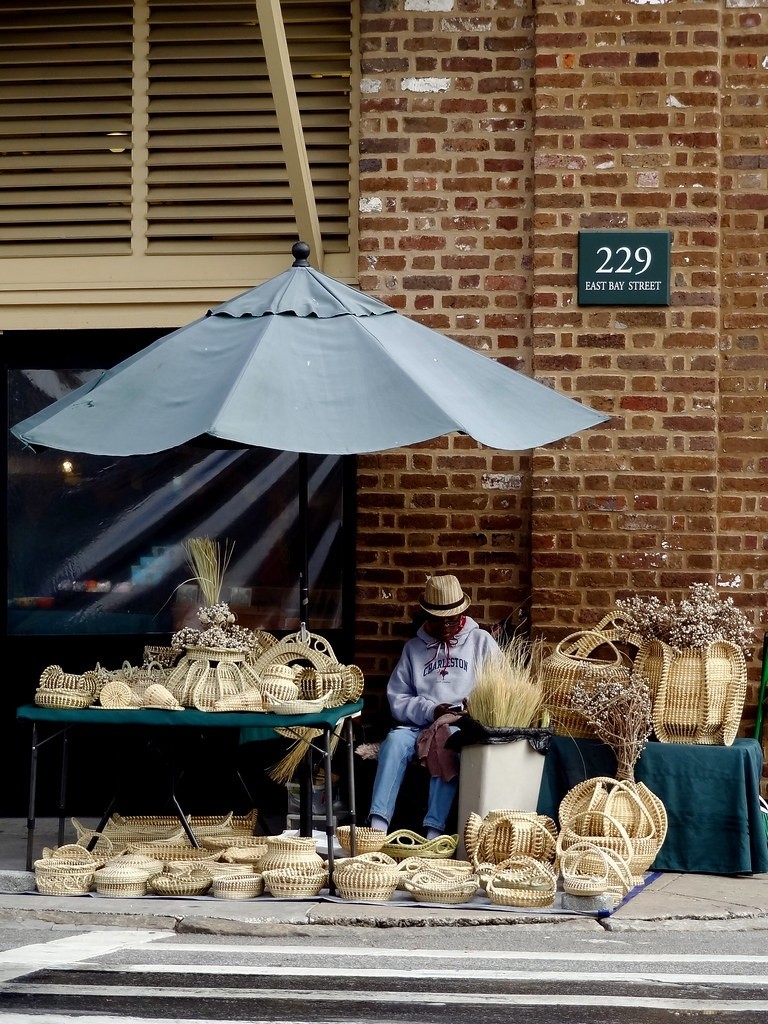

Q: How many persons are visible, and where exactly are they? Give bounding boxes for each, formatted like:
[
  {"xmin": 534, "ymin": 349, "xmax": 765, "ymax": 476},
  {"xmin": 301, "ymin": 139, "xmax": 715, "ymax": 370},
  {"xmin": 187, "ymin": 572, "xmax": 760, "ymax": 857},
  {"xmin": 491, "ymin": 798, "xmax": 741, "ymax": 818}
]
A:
[{"xmin": 366, "ymin": 573, "xmax": 513, "ymax": 841}]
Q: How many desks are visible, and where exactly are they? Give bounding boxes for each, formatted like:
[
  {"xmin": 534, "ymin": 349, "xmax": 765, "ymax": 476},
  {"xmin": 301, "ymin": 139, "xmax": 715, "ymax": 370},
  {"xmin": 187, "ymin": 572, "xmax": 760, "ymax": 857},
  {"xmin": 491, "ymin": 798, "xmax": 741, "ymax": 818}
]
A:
[
  {"xmin": 536, "ymin": 734, "xmax": 767, "ymax": 875},
  {"xmin": 6, "ymin": 609, "xmax": 157, "ymax": 636},
  {"xmin": 14, "ymin": 699, "xmax": 367, "ymax": 897}
]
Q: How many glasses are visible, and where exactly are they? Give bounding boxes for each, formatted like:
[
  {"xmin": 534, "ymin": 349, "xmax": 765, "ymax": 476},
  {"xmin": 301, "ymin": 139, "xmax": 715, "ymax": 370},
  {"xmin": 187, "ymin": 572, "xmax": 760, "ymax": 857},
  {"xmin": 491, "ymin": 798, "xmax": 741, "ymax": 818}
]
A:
[{"xmin": 428, "ymin": 615, "xmax": 462, "ymax": 627}]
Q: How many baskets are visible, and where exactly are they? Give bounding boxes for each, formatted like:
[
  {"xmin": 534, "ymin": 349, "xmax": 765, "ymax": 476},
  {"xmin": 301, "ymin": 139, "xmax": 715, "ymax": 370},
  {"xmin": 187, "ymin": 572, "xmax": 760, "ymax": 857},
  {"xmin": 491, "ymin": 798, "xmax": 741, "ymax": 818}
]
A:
[
  {"xmin": 33, "ymin": 776, "xmax": 668, "ymax": 910},
  {"xmin": 34, "ymin": 628, "xmax": 365, "ymax": 715},
  {"xmin": 538, "ymin": 610, "xmax": 748, "ymax": 747}
]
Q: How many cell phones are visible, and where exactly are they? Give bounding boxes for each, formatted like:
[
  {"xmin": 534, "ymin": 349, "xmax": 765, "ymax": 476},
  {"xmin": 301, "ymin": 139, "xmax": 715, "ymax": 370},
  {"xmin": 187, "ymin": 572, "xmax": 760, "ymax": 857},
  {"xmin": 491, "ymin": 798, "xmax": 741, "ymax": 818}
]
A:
[{"xmin": 448, "ymin": 704, "xmax": 461, "ymax": 713}]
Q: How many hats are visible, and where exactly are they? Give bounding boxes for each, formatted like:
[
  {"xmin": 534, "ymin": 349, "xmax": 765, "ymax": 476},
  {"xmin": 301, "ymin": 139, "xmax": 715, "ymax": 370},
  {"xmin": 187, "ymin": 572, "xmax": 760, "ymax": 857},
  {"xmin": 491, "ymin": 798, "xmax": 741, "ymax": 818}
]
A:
[{"xmin": 418, "ymin": 574, "xmax": 472, "ymax": 616}]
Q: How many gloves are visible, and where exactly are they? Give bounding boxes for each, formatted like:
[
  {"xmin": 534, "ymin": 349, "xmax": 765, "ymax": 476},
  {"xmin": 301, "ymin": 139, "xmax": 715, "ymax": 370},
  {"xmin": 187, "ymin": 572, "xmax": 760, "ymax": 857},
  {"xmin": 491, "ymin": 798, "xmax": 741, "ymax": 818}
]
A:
[{"xmin": 433, "ymin": 703, "xmax": 458, "ymax": 721}]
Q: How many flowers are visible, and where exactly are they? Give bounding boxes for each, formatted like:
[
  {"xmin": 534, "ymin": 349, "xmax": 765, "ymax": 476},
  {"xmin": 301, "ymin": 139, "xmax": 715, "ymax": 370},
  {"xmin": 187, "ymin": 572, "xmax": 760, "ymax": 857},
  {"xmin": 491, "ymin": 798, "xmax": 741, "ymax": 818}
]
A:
[
  {"xmin": 614, "ymin": 581, "xmax": 755, "ymax": 656},
  {"xmin": 566, "ymin": 660, "xmax": 653, "ymax": 786},
  {"xmin": 172, "ymin": 604, "xmax": 261, "ymax": 655}
]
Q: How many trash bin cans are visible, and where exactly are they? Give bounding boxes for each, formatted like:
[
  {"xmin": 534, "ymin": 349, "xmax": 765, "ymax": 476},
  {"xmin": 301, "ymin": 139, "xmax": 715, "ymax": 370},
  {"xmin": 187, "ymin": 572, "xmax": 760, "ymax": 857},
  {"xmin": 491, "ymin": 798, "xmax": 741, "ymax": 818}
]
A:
[{"xmin": 455, "ymin": 717, "xmax": 555, "ymax": 863}]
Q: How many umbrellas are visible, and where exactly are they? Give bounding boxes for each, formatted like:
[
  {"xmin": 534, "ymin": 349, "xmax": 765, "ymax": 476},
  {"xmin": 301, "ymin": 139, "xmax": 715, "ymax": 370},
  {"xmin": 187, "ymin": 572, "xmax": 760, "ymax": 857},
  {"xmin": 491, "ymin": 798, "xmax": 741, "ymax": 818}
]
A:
[{"xmin": 11, "ymin": 238, "xmax": 617, "ymax": 857}]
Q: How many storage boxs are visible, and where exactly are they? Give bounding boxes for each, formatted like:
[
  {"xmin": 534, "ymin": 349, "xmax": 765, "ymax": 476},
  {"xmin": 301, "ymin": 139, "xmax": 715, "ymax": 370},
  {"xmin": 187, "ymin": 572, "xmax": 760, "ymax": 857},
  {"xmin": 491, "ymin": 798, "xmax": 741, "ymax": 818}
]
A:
[
  {"xmin": 287, "ymin": 810, "xmax": 350, "ymax": 836},
  {"xmin": 284, "ymin": 782, "xmax": 340, "ymax": 816}
]
